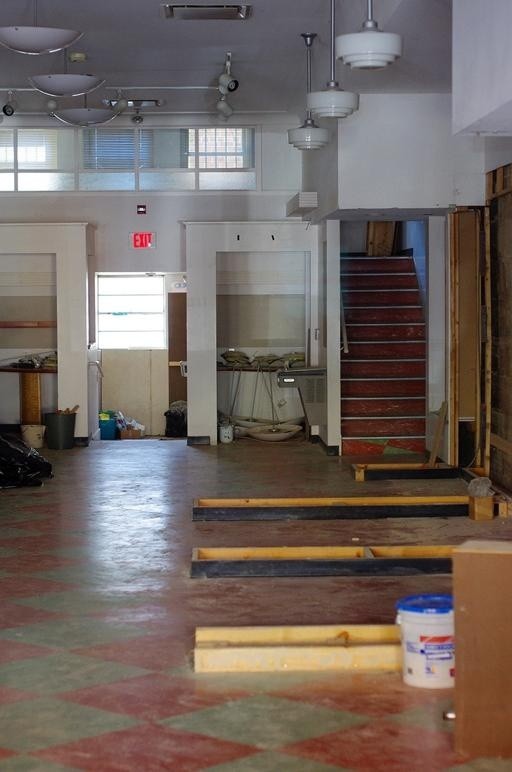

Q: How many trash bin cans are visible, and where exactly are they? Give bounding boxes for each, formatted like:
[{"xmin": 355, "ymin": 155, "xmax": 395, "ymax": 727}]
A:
[
  {"xmin": 98, "ymin": 409, "xmax": 117, "ymax": 440},
  {"xmin": 164, "ymin": 410, "xmax": 187, "ymax": 437}
]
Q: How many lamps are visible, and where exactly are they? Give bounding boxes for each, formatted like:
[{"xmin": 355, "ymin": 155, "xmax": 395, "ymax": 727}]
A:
[
  {"xmin": 1, "ymin": 1, "xmax": 118, "ymax": 130},
  {"xmin": 286, "ymin": 1, "xmax": 403, "ymax": 151}
]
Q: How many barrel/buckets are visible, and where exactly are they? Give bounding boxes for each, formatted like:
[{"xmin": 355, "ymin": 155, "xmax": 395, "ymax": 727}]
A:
[
  {"xmin": 395, "ymin": 591, "xmax": 457, "ymax": 690},
  {"xmin": 20, "ymin": 425, "xmax": 47, "ymax": 448}
]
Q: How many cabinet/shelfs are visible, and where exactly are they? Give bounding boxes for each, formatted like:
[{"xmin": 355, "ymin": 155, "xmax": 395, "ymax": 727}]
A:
[{"xmin": 0, "ymin": 320, "xmax": 57, "ymax": 374}]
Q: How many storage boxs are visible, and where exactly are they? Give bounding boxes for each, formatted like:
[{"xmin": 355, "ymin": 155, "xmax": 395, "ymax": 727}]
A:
[{"xmin": 99, "ymin": 409, "xmax": 141, "ymax": 439}]
